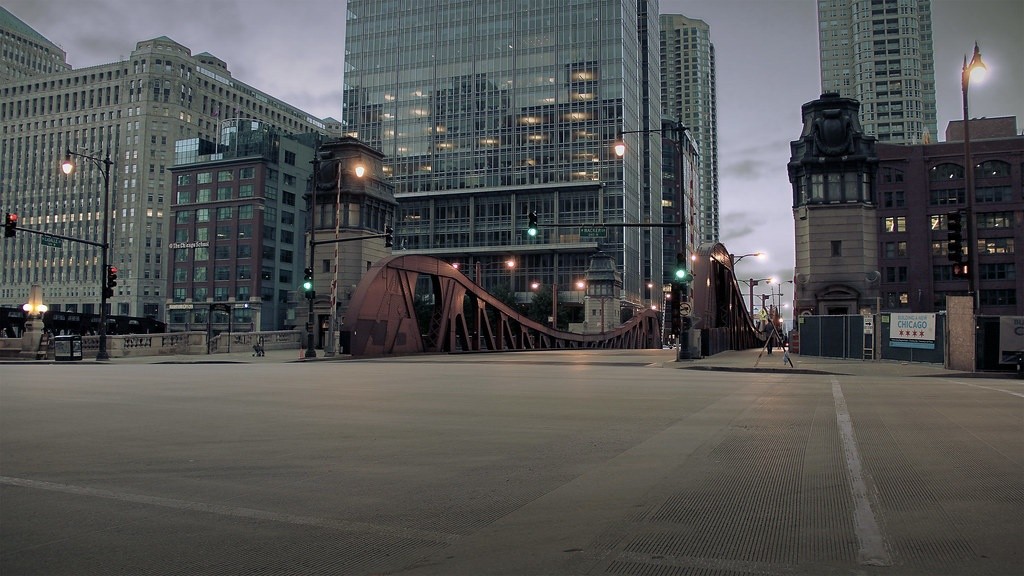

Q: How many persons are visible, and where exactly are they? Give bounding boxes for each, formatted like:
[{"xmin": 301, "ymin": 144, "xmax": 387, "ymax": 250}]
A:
[
  {"xmin": 43, "ymin": 329, "xmax": 55, "ymax": 349},
  {"xmin": 776, "ymin": 328, "xmax": 783, "ymax": 349},
  {"xmin": 765, "ymin": 318, "xmax": 773, "ymax": 354}
]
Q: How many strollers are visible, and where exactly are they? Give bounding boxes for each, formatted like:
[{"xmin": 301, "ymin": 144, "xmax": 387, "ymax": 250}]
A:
[{"xmin": 252, "ymin": 336, "xmax": 265, "ymax": 357}]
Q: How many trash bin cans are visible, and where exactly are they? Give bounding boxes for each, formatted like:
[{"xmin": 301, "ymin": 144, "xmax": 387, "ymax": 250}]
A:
[{"xmin": 52, "ymin": 334, "xmax": 84, "ymax": 361}]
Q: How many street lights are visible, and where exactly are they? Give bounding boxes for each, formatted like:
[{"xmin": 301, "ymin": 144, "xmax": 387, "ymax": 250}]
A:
[
  {"xmin": 62, "ymin": 146, "xmax": 114, "ymax": 361},
  {"xmin": 530, "ymin": 281, "xmax": 587, "ymax": 329},
  {"xmin": 959, "ymin": 38, "xmax": 988, "ymax": 313},
  {"xmin": 647, "ymin": 282, "xmax": 671, "ymax": 312},
  {"xmin": 450, "ymin": 258, "xmax": 517, "ymax": 287},
  {"xmin": 612, "ymin": 111, "xmax": 690, "ymax": 360},
  {"xmin": 305, "ymin": 137, "xmax": 366, "ymax": 357},
  {"xmin": 584, "ymin": 294, "xmax": 628, "ymax": 333},
  {"xmin": 738, "ymin": 277, "xmax": 777, "ymax": 324},
  {"xmin": 766, "ymin": 280, "xmax": 793, "ymax": 330},
  {"xmin": 741, "ymin": 293, "xmax": 784, "ymax": 331},
  {"xmin": 729, "ymin": 252, "xmax": 765, "ymax": 273}
]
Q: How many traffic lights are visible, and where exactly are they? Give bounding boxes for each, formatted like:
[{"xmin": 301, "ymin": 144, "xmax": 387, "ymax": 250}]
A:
[
  {"xmin": 107, "ymin": 264, "xmax": 118, "ymax": 287},
  {"xmin": 946, "ymin": 207, "xmax": 964, "ymax": 263},
  {"xmin": 527, "ymin": 210, "xmax": 539, "ymax": 237},
  {"xmin": 385, "ymin": 226, "xmax": 394, "ymax": 247},
  {"xmin": 303, "ymin": 267, "xmax": 313, "ymax": 291},
  {"xmin": 105, "ymin": 287, "xmax": 113, "ymax": 298},
  {"xmin": 3, "ymin": 210, "xmax": 19, "ymax": 238},
  {"xmin": 952, "ymin": 261, "xmax": 969, "ymax": 280},
  {"xmin": 670, "ymin": 279, "xmax": 682, "ymax": 335},
  {"xmin": 675, "ymin": 252, "xmax": 694, "ymax": 282},
  {"xmin": 304, "ymin": 290, "xmax": 316, "ymax": 299}
]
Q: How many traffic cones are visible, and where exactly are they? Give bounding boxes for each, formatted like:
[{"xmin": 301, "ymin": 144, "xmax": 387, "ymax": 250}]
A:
[{"xmin": 299, "ymin": 347, "xmax": 305, "ymax": 359}]
